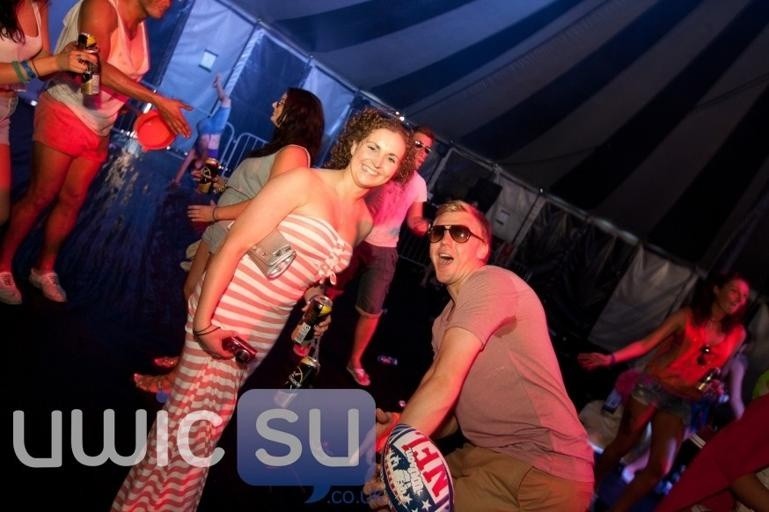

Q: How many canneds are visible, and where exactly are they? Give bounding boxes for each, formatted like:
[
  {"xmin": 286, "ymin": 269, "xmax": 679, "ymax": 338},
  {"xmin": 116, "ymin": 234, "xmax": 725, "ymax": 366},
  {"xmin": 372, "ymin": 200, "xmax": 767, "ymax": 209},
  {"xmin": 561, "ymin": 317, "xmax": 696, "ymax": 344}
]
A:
[
  {"xmin": 196, "ymin": 158, "xmax": 218, "ymax": 195},
  {"xmin": 77, "ymin": 32, "xmax": 95, "ymax": 50},
  {"xmin": 274, "ymin": 356, "xmax": 320, "ymax": 408},
  {"xmin": 290, "ymin": 296, "xmax": 333, "ymax": 346},
  {"xmin": 82, "ymin": 49, "xmax": 101, "ymax": 95},
  {"xmin": 695, "ymin": 367, "xmax": 717, "ymax": 391},
  {"xmin": 602, "ymin": 389, "xmax": 622, "ymax": 416}
]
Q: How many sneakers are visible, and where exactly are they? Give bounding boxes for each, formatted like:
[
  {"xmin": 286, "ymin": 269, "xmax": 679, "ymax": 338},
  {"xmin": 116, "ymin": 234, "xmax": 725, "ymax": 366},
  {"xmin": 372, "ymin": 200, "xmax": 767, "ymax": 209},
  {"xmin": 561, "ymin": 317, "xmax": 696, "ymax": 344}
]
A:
[
  {"xmin": 347, "ymin": 361, "xmax": 371, "ymax": 386},
  {"xmin": 0, "ymin": 271, "xmax": 21, "ymax": 306},
  {"xmin": 28, "ymin": 269, "xmax": 67, "ymax": 302}
]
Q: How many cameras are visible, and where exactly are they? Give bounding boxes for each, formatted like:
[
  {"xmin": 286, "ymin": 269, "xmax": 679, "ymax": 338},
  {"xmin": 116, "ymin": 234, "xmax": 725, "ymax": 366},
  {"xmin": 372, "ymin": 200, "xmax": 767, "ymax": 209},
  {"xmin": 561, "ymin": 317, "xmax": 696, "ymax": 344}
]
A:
[{"xmin": 223, "ymin": 336, "xmax": 257, "ymax": 364}]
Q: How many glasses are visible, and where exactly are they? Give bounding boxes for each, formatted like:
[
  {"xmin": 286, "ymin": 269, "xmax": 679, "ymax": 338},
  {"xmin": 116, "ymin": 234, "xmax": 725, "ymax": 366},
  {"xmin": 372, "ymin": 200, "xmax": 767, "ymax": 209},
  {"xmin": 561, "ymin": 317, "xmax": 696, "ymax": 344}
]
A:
[
  {"xmin": 413, "ymin": 138, "xmax": 432, "ymax": 153},
  {"xmin": 698, "ymin": 346, "xmax": 709, "ymax": 364},
  {"xmin": 428, "ymin": 224, "xmax": 484, "ymax": 244}
]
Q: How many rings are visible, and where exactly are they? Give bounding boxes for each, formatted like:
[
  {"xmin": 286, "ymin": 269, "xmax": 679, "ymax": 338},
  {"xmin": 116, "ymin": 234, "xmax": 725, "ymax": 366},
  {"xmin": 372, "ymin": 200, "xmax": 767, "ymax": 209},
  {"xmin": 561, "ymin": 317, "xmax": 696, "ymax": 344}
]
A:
[{"xmin": 78, "ymin": 58, "xmax": 83, "ymax": 64}]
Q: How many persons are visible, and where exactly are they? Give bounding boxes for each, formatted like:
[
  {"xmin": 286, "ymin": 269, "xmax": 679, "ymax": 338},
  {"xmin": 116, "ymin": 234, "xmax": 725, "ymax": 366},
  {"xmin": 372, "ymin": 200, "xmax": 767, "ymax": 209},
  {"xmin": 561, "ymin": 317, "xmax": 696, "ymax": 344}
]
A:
[
  {"xmin": 348, "ymin": 200, "xmax": 595, "ymax": 511},
  {"xmin": 571, "ymin": 272, "xmax": 752, "ymax": 510},
  {"xmin": 170, "ymin": 76, "xmax": 233, "ymax": 191},
  {"xmin": 106, "ymin": 105, "xmax": 415, "ymax": 511},
  {"xmin": 650, "ymin": 394, "xmax": 769, "ymax": 512},
  {"xmin": 0, "ymin": 0, "xmax": 98, "ymax": 227},
  {"xmin": 0, "ymin": 0, "xmax": 195, "ymax": 305},
  {"xmin": 321, "ymin": 128, "xmax": 437, "ymax": 386},
  {"xmin": 134, "ymin": 86, "xmax": 326, "ymax": 393}
]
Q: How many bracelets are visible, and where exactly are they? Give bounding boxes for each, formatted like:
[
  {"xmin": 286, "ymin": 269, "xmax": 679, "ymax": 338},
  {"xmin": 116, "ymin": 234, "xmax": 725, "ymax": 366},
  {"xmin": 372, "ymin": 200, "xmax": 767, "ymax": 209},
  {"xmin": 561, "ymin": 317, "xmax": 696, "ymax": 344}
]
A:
[
  {"xmin": 212, "ymin": 207, "xmax": 220, "ymax": 223},
  {"xmin": 193, "ymin": 322, "xmax": 222, "ymax": 336},
  {"xmin": 11, "ymin": 59, "xmax": 41, "ymax": 82},
  {"xmin": 608, "ymin": 352, "xmax": 617, "ymax": 363}
]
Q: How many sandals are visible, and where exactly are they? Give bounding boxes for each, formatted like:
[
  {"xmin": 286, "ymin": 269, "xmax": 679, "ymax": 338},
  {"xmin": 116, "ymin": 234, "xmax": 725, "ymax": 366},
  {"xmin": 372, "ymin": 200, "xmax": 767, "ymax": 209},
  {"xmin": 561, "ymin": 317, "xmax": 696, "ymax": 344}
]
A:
[{"xmin": 135, "ymin": 357, "xmax": 178, "ymax": 395}]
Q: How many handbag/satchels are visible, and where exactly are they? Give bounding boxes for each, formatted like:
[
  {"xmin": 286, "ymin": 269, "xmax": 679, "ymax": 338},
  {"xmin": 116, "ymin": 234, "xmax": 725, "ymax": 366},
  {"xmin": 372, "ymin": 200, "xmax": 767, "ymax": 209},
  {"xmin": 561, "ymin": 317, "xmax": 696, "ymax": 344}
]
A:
[{"xmin": 227, "ymin": 219, "xmax": 295, "ymax": 280}]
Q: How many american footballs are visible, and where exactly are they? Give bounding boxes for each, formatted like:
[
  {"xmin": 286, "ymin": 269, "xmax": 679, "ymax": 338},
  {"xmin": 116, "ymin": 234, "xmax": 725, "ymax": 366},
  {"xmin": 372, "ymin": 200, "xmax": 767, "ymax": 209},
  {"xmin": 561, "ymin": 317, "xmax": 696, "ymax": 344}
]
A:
[{"xmin": 380, "ymin": 422, "xmax": 455, "ymax": 512}]
